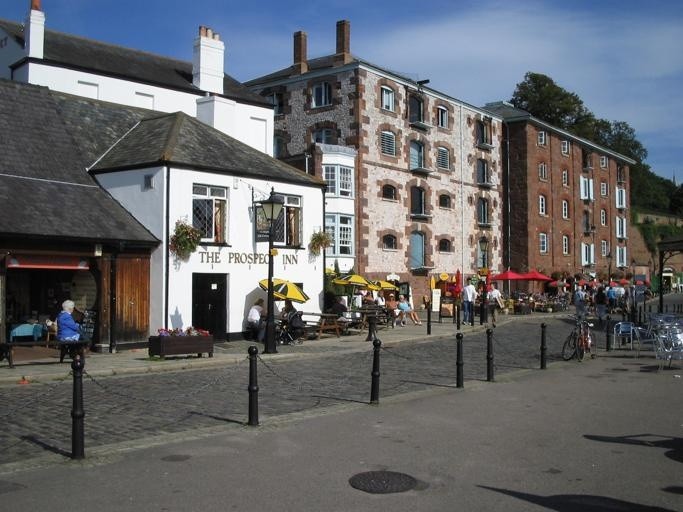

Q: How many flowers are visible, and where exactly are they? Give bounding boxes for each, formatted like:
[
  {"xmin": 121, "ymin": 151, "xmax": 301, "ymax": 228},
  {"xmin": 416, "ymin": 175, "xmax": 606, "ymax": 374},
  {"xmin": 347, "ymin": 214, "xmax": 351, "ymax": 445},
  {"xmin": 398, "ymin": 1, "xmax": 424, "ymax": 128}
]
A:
[{"xmin": 157, "ymin": 326, "xmax": 211, "ymax": 336}]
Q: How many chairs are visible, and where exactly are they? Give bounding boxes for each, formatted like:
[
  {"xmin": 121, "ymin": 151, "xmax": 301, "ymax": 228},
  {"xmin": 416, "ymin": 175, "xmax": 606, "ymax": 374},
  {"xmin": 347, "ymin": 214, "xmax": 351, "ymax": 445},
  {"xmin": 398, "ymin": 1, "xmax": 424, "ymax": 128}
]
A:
[
  {"xmin": 614, "ymin": 312, "xmax": 683, "ymax": 372},
  {"xmin": 43, "ymin": 317, "xmax": 57, "ymax": 348}
]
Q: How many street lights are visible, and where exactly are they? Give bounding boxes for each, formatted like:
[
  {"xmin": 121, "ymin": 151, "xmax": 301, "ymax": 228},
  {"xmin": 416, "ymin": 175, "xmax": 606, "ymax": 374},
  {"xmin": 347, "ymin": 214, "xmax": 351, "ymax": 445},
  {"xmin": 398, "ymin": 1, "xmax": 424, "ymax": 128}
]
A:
[
  {"xmin": 260, "ymin": 188, "xmax": 286, "ymax": 353},
  {"xmin": 479, "ymin": 234, "xmax": 489, "ymax": 324}
]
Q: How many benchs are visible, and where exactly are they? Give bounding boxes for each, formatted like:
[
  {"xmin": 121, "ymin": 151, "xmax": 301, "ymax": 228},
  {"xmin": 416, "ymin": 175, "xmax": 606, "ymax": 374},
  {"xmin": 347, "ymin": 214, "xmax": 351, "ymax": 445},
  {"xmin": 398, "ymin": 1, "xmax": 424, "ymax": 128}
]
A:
[
  {"xmin": 246, "ymin": 303, "xmax": 407, "ymax": 340},
  {"xmin": 0, "ymin": 340, "xmax": 90, "ymax": 368}
]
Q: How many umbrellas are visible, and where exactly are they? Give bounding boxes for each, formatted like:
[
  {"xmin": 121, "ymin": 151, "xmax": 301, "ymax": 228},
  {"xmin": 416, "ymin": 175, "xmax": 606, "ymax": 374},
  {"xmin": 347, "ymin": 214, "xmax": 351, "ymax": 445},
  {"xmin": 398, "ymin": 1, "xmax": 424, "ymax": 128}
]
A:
[
  {"xmin": 549, "ymin": 276, "xmax": 649, "ymax": 289},
  {"xmin": 258, "ymin": 278, "xmax": 310, "ymax": 313},
  {"xmin": 454, "ymin": 270, "xmax": 462, "ymax": 298},
  {"xmin": 485, "ymin": 272, "xmax": 493, "ymax": 292},
  {"xmin": 330, "ymin": 275, "xmax": 369, "ymax": 305},
  {"xmin": 365, "ymin": 279, "xmax": 400, "ymax": 303},
  {"xmin": 520, "ymin": 270, "xmax": 554, "ymax": 292},
  {"xmin": 493, "ymin": 270, "xmax": 526, "ymax": 299}
]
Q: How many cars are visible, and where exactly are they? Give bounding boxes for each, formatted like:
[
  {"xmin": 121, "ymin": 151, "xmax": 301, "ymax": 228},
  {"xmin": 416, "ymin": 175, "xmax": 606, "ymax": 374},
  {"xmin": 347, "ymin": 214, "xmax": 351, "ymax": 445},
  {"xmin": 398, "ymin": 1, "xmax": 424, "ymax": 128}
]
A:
[{"xmin": 606, "ymin": 287, "xmax": 638, "ymax": 313}]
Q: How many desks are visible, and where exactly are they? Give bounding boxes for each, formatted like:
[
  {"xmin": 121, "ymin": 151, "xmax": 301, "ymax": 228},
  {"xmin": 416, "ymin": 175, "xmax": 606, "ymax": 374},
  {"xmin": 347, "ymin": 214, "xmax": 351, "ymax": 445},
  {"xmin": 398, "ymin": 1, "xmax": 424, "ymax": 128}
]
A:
[{"xmin": 6, "ymin": 323, "xmax": 44, "ymax": 342}]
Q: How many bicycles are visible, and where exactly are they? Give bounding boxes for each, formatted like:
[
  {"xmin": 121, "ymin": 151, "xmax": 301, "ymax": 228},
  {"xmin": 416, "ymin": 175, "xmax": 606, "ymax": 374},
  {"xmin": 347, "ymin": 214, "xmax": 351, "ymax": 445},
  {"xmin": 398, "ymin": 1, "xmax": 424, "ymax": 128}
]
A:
[
  {"xmin": 561, "ymin": 312, "xmax": 597, "ymax": 362},
  {"xmin": 560, "ymin": 298, "xmax": 569, "ymax": 311},
  {"xmin": 609, "ymin": 296, "xmax": 618, "ymax": 314},
  {"xmin": 582, "ymin": 297, "xmax": 599, "ymax": 317}
]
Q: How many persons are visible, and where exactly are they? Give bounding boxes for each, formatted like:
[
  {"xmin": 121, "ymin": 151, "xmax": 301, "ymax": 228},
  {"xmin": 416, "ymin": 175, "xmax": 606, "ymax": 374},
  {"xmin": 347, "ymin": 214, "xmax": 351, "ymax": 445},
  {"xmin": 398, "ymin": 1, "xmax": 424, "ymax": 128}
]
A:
[
  {"xmin": 360, "ymin": 292, "xmax": 375, "ymax": 304},
  {"xmin": 573, "ymin": 288, "xmax": 586, "ymax": 319},
  {"xmin": 594, "ymin": 287, "xmax": 607, "ymax": 319},
  {"xmin": 486, "ymin": 282, "xmax": 502, "ymax": 327},
  {"xmin": 246, "ymin": 298, "xmax": 267, "ymax": 342},
  {"xmin": 461, "ymin": 277, "xmax": 477, "ymax": 327},
  {"xmin": 384, "ymin": 294, "xmax": 405, "ymax": 328},
  {"xmin": 330, "ymin": 296, "xmax": 351, "ymax": 335},
  {"xmin": 606, "ymin": 286, "xmax": 616, "ymax": 316},
  {"xmin": 375, "ymin": 291, "xmax": 385, "ymax": 306},
  {"xmin": 55, "ymin": 300, "xmax": 92, "ymax": 355},
  {"xmin": 396, "ymin": 295, "xmax": 422, "ymax": 325},
  {"xmin": 279, "ymin": 301, "xmax": 297, "ymax": 330}
]
